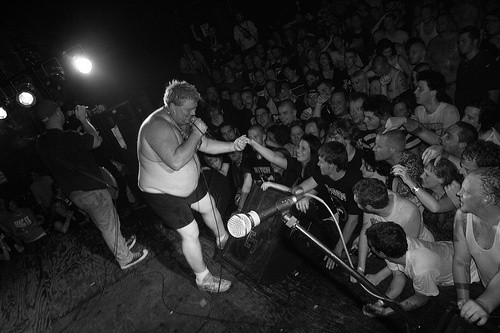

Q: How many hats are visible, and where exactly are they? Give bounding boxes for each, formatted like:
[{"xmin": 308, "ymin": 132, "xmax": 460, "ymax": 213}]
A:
[
  {"xmin": 307, "ymin": 87, "xmax": 319, "ymax": 95},
  {"xmin": 37, "ymin": 98, "xmax": 62, "ymax": 121}
]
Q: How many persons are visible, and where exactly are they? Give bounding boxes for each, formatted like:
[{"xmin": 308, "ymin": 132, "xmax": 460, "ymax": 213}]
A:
[{"xmin": 0, "ymin": 0, "xmax": 500, "ymax": 333}]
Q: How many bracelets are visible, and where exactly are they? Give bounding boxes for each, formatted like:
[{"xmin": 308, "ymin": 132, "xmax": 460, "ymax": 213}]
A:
[{"xmin": 411, "ymin": 185, "xmax": 420, "ymax": 193}]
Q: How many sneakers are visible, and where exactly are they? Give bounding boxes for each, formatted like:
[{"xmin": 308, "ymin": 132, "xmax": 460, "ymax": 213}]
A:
[
  {"xmin": 217, "ymin": 235, "xmax": 229, "ymax": 250},
  {"xmin": 121, "ymin": 249, "xmax": 148, "ymax": 269},
  {"xmin": 195, "ymin": 274, "xmax": 232, "ymax": 293},
  {"xmin": 127, "ymin": 236, "xmax": 137, "ymax": 250}
]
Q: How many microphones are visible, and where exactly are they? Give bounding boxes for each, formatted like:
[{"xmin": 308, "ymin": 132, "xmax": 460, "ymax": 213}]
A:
[
  {"xmin": 226, "ymin": 193, "xmax": 306, "ymax": 239},
  {"xmin": 65, "ymin": 110, "xmax": 89, "ymax": 116},
  {"xmin": 189, "ymin": 115, "xmax": 215, "ymax": 140}
]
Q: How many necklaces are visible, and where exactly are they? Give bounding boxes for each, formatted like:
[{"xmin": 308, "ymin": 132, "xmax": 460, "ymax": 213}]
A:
[{"xmin": 165, "ymin": 107, "xmax": 189, "ymax": 140}]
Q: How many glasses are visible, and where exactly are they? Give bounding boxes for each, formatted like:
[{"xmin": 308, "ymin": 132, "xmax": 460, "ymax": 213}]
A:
[{"xmin": 307, "ymin": 94, "xmax": 319, "ymax": 99}]
